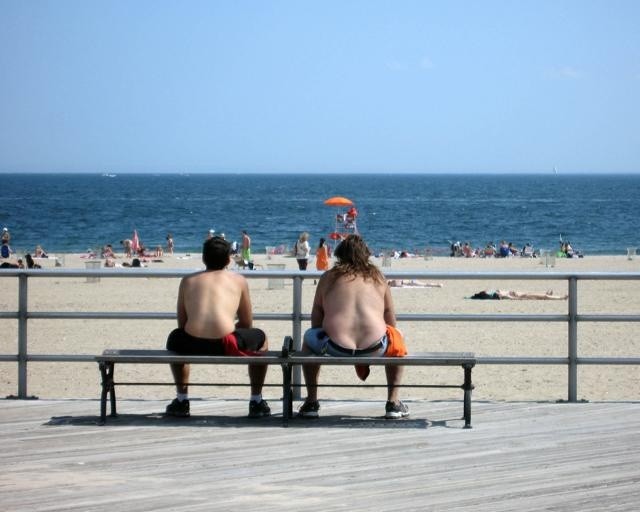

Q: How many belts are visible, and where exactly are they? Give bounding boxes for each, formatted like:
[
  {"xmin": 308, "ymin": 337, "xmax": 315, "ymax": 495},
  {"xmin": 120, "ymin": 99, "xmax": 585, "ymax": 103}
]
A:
[{"xmin": 329, "ymin": 340, "xmax": 383, "ymax": 354}]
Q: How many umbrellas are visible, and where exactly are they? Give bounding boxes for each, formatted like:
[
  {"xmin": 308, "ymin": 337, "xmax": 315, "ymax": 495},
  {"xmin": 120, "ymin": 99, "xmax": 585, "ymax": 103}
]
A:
[
  {"xmin": 323, "ymin": 196, "xmax": 353, "ymax": 215},
  {"xmin": 131, "ymin": 229, "xmax": 140, "ymax": 256}
]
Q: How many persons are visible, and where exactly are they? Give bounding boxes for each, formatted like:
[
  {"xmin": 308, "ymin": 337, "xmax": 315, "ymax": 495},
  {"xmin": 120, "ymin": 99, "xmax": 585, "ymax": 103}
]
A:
[
  {"xmin": 0, "ymin": 227, "xmax": 11, "ymax": 244},
  {"xmin": 80, "ymin": 249, "xmax": 98, "ymax": 260},
  {"xmin": 240, "ymin": 230, "xmax": 257, "ymax": 270},
  {"xmin": 165, "ymin": 235, "xmax": 272, "ymax": 418},
  {"xmin": 17, "ymin": 258, "xmax": 25, "ymax": 269},
  {"xmin": 402, "ymin": 251, "xmax": 424, "ymax": 258},
  {"xmin": 105, "ymin": 253, "xmax": 125, "ymax": 268},
  {"xmin": 298, "ymin": 233, "xmax": 411, "ymax": 420},
  {"xmin": 220, "ymin": 233, "xmax": 225, "ymax": 241},
  {"xmin": 208, "ymin": 229, "xmax": 215, "ymax": 240},
  {"xmin": 137, "ymin": 242, "xmax": 157, "ymax": 258},
  {"xmin": 476, "ymin": 288, "xmax": 527, "ymax": 299},
  {"xmin": 390, "ymin": 248, "xmax": 401, "ymax": 260},
  {"xmin": 119, "ymin": 239, "xmax": 133, "ymax": 259},
  {"xmin": 313, "ymin": 237, "xmax": 331, "ymax": 285},
  {"xmin": 101, "ymin": 244, "xmax": 118, "ymax": 259},
  {"xmin": 33, "ymin": 245, "xmax": 51, "ymax": 258},
  {"xmin": 24, "ymin": 253, "xmax": 42, "ymax": 269},
  {"xmin": 0, "ymin": 241, "xmax": 14, "ymax": 259},
  {"xmin": 387, "ymin": 278, "xmax": 444, "ymax": 288},
  {"xmin": 273, "ymin": 240, "xmax": 288, "ymax": 255},
  {"xmin": 509, "ymin": 290, "xmax": 570, "ymax": 301},
  {"xmin": 156, "ymin": 245, "xmax": 165, "ymax": 258},
  {"xmin": 493, "ymin": 288, "xmax": 554, "ymax": 299},
  {"xmin": 293, "ymin": 231, "xmax": 312, "ymax": 271},
  {"xmin": 446, "ymin": 235, "xmax": 585, "ymax": 260},
  {"xmin": 166, "ymin": 234, "xmax": 175, "ymax": 255}
]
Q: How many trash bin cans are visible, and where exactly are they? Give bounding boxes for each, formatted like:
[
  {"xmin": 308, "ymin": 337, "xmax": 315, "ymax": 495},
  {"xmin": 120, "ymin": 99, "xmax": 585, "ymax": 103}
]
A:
[
  {"xmin": 266, "ymin": 263, "xmax": 286, "ymax": 291},
  {"xmin": 84, "ymin": 261, "xmax": 102, "ymax": 284}
]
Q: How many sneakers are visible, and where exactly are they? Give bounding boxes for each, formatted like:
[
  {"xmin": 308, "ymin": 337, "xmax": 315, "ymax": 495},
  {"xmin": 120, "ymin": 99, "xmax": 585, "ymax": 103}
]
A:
[
  {"xmin": 166, "ymin": 398, "xmax": 190, "ymax": 417},
  {"xmin": 386, "ymin": 400, "xmax": 409, "ymax": 419},
  {"xmin": 249, "ymin": 399, "xmax": 270, "ymax": 418},
  {"xmin": 300, "ymin": 400, "xmax": 320, "ymax": 417}
]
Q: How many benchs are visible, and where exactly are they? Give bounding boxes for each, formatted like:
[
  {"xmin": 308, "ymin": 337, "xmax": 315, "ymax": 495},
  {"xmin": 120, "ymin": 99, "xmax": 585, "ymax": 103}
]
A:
[{"xmin": 94, "ymin": 336, "xmax": 477, "ymax": 428}]
